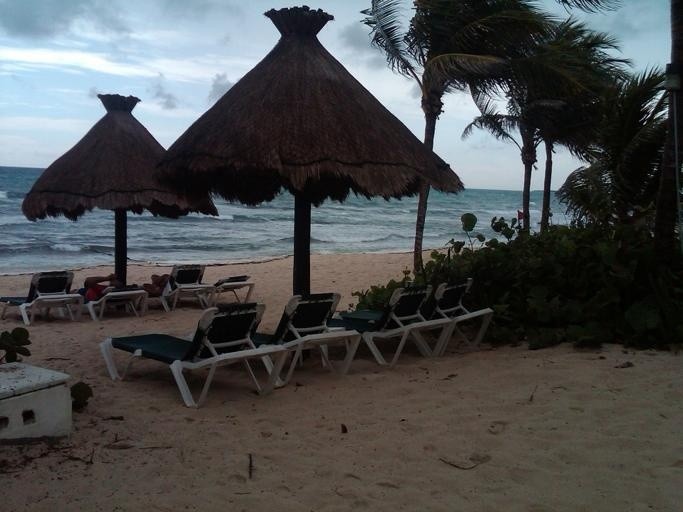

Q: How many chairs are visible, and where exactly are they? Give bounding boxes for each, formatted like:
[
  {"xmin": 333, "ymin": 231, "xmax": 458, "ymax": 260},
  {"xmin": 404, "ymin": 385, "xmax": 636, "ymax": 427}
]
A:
[
  {"xmin": 231, "ymin": 292, "xmax": 361, "ymax": 389},
  {"xmin": 99, "ymin": 302, "xmax": 288, "ymax": 409},
  {"xmin": 2, "ymin": 264, "xmax": 216, "ymax": 327},
  {"xmin": 326, "ymin": 284, "xmax": 452, "ymax": 369},
  {"xmin": 341, "ymin": 278, "xmax": 495, "ymax": 360}
]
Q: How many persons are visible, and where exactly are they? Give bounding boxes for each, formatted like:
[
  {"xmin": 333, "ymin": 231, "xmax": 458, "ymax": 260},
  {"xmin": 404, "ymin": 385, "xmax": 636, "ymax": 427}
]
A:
[
  {"xmin": 70, "ymin": 274, "xmax": 122, "ymax": 302},
  {"xmin": 144, "ymin": 273, "xmax": 170, "ymax": 294}
]
